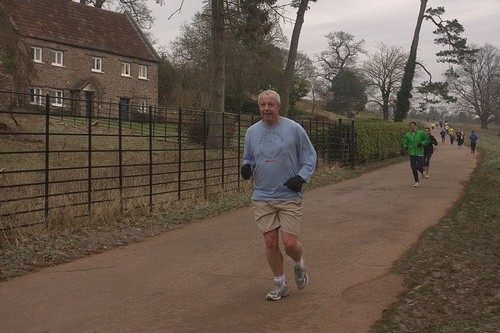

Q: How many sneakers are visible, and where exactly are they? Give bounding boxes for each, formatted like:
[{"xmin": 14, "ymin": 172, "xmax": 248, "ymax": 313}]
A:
[
  {"xmin": 422, "ymin": 170, "xmax": 430, "ymax": 180},
  {"xmin": 413, "ymin": 181, "xmax": 421, "ymax": 187},
  {"xmin": 294, "ymin": 265, "xmax": 309, "ymax": 289},
  {"xmin": 266, "ymin": 281, "xmax": 290, "ymax": 300}
]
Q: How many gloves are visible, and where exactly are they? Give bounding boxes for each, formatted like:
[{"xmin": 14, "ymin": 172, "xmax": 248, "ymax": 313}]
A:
[
  {"xmin": 240, "ymin": 164, "xmax": 252, "ymax": 180},
  {"xmin": 417, "ymin": 142, "xmax": 423, "ymax": 148},
  {"xmin": 403, "ymin": 148, "xmax": 408, "ymax": 154},
  {"xmin": 283, "ymin": 174, "xmax": 305, "ymax": 193}
]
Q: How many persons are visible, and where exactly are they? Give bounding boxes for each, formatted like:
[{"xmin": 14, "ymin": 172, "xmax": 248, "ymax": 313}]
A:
[
  {"xmin": 423, "ymin": 127, "xmax": 438, "ymax": 178},
  {"xmin": 240, "ymin": 89, "xmax": 317, "ymax": 301},
  {"xmin": 402, "ymin": 121, "xmax": 430, "ymax": 187},
  {"xmin": 469, "ymin": 131, "xmax": 478, "ymax": 154},
  {"xmin": 432, "ymin": 119, "xmax": 465, "ymax": 149}
]
